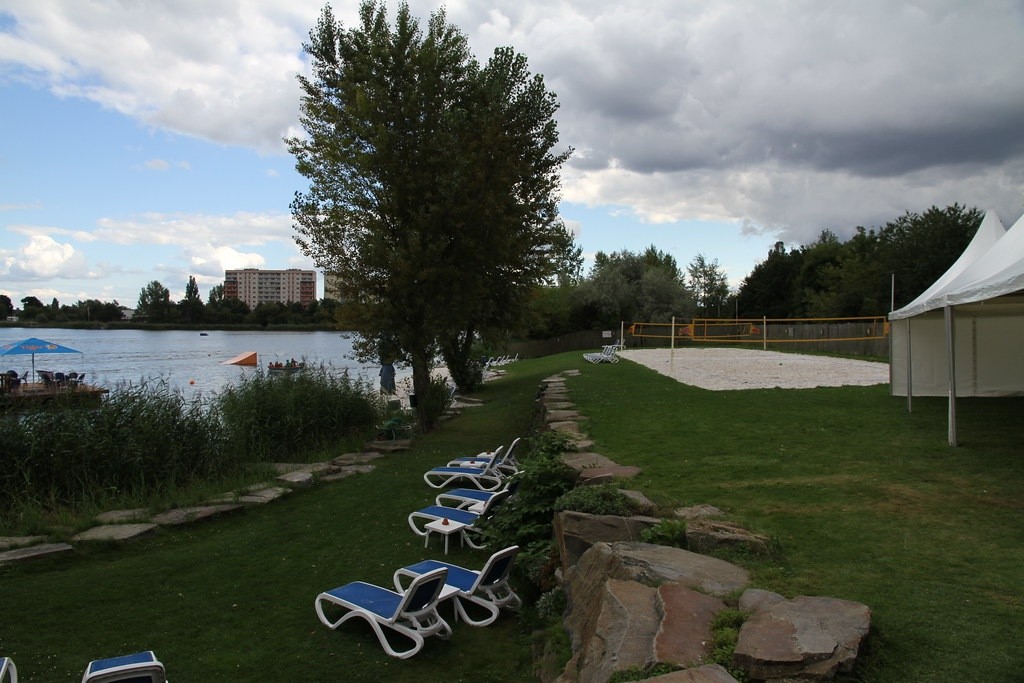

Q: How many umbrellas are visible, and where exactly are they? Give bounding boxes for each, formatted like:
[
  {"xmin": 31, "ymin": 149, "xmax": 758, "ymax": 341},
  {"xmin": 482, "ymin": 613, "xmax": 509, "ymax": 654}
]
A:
[{"xmin": 0, "ymin": 338, "xmax": 83, "ymax": 388}]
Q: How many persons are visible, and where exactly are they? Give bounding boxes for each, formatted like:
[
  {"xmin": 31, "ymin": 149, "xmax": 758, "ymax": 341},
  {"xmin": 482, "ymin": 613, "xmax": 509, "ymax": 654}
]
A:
[
  {"xmin": 275, "ymin": 358, "xmax": 296, "ymax": 367},
  {"xmin": 269, "ymin": 362, "xmax": 274, "ymax": 368}
]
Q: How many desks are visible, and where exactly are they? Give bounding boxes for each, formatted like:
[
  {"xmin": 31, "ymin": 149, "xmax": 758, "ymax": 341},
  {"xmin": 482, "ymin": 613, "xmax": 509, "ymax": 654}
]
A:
[
  {"xmin": 0, "ymin": 373, "xmax": 12, "ymax": 391},
  {"xmin": 460, "ymin": 462, "xmax": 485, "ymax": 468},
  {"xmin": 476, "ymin": 451, "xmax": 495, "ymax": 458},
  {"xmin": 404, "ymin": 582, "xmax": 460, "ymax": 608},
  {"xmin": 468, "ymin": 501, "xmax": 486, "ymax": 514},
  {"xmin": 424, "ymin": 517, "xmax": 466, "ymax": 554}
]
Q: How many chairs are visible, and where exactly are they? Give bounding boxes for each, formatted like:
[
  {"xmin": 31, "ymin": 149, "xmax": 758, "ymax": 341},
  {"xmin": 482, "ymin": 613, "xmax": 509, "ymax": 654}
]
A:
[
  {"xmin": 447, "ymin": 438, "xmax": 520, "ymax": 479},
  {"xmin": 448, "ymin": 386, "xmax": 457, "ymax": 406},
  {"xmin": 393, "ymin": 545, "xmax": 525, "ymax": 628},
  {"xmin": 315, "ymin": 567, "xmax": 447, "ymax": 660},
  {"xmin": 583, "ymin": 339, "xmax": 626, "ymax": 364},
  {"xmin": 408, "ymin": 490, "xmax": 512, "ymax": 549},
  {"xmin": 435, "ymin": 470, "xmax": 526, "ymax": 512},
  {"xmin": 6, "ymin": 370, "xmax": 86, "ymax": 389},
  {"xmin": 482, "ymin": 353, "xmax": 518, "ymax": 380},
  {"xmin": 81, "ymin": 650, "xmax": 169, "ymax": 683},
  {"xmin": 424, "ymin": 445, "xmax": 503, "ymax": 491}
]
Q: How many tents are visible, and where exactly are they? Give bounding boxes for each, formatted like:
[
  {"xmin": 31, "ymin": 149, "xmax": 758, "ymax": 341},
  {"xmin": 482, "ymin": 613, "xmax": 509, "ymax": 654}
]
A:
[{"xmin": 888, "ymin": 209, "xmax": 1024, "ymax": 445}]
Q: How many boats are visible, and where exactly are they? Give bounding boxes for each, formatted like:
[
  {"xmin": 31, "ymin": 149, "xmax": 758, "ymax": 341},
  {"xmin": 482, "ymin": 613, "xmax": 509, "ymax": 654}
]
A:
[
  {"xmin": 0, "ymin": 368, "xmax": 111, "ymax": 412},
  {"xmin": 268, "ymin": 366, "xmax": 302, "ymax": 374}
]
